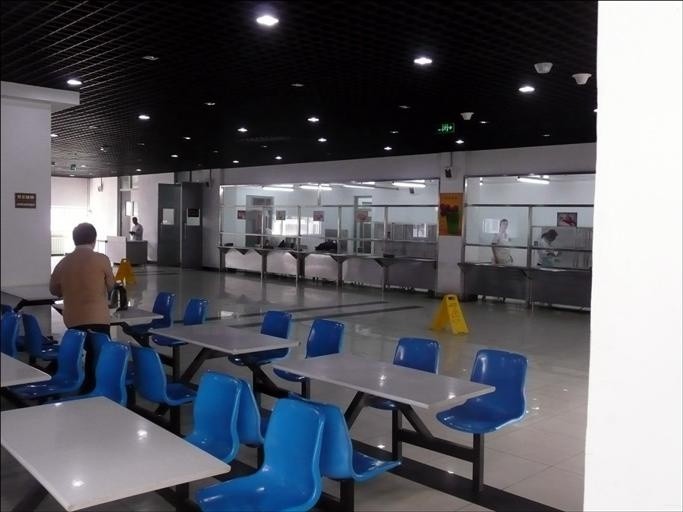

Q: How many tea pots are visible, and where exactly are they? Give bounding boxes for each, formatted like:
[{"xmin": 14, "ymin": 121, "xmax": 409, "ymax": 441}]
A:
[{"xmin": 110, "ymin": 279, "xmax": 127, "ymax": 311}]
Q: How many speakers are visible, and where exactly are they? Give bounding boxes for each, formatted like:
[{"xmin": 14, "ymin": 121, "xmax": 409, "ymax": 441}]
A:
[{"xmin": 444, "ymin": 167, "xmax": 452, "ymax": 178}]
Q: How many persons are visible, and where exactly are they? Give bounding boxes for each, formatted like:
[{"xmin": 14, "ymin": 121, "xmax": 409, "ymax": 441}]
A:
[
  {"xmin": 48, "ymin": 221, "xmax": 117, "ymax": 342},
  {"xmin": 258, "ymin": 226, "xmax": 272, "ymax": 246},
  {"xmin": 537, "ymin": 230, "xmax": 560, "ymax": 270},
  {"xmin": 128, "ymin": 216, "xmax": 143, "ymax": 241},
  {"xmin": 492, "ymin": 219, "xmax": 515, "ymax": 264}
]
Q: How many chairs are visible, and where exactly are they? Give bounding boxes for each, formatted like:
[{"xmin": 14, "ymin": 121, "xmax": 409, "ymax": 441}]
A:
[
  {"xmin": 170, "ymin": 372, "xmax": 241, "ymax": 509},
  {"xmin": 236, "ymin": 378, "xmax": 268, "ymax": 471},
  {"xmin": 291, "ymin": 394, "xmax": 402, "ymax": 511},
  {"xmin": 436, "ymin": 350, "xmax": 526, "ymax": 497},
  {"xmin": 366, "ymin": 337, "xmax": 440, "ymax": 466},
  {"xmin": 194, "ymin": 399, "xmax": 322, "ymax": 511},
  {"xmin": 0, "ymin": 291, "xmax": 345, "ymax": 431}
]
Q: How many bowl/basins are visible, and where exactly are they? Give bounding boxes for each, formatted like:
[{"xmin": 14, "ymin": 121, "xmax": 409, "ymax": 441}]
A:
[{"xmin": 107, "ymin": 306, "xmax": 119, "ymax": 317}]
